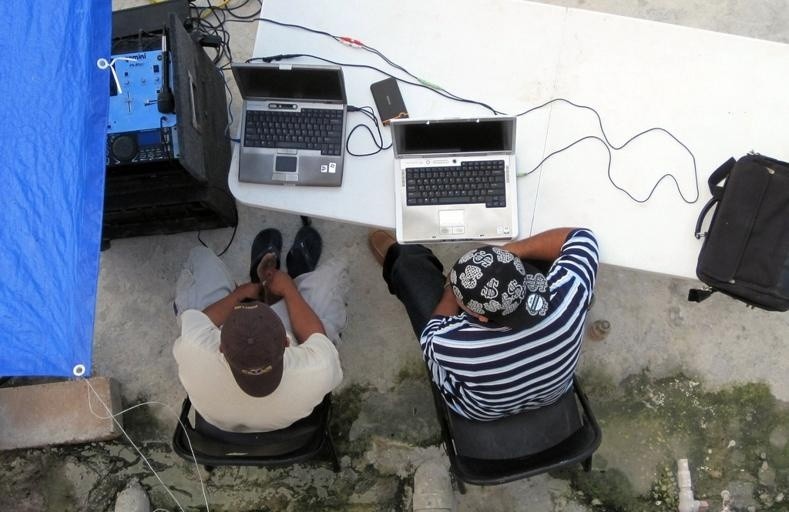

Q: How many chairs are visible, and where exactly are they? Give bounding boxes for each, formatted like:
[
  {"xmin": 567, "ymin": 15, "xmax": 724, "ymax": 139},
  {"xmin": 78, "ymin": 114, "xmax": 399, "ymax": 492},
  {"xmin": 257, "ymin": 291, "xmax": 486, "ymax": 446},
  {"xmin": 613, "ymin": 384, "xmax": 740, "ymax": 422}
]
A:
[
  {"xmin": 171, "ymin": 391, "xmax": 342, "ymax": 476},
  {"xmin": 424, "ymin": 358, "xmax": 601, "ymax": 494}
]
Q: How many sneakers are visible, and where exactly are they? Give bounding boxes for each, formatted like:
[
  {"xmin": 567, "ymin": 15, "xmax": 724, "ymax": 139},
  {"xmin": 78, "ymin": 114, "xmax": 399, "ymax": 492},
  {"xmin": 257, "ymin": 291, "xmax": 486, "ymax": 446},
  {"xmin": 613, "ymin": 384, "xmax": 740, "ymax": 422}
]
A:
[
  {"xmin": 248, "ymin": 228, "xmax": 282, "ymax": 285},
  {"xmin": 370, "ymin": 228, "xmax": 398, "ymax": 266},
  {"xmin": 287, "ymin": 227, "xmax": 323, "ymax": 279}
]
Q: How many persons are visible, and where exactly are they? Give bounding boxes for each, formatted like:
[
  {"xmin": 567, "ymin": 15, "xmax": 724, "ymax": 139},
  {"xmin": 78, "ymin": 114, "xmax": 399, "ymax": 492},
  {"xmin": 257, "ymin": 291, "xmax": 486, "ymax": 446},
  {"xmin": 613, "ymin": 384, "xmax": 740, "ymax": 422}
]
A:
[
  {"xmin": 172, "ymin": 225, "xmax": 352, "ymax": 434},
  {"xmin": 366, "ymin": 225, "xmax": 599, "ymax": 425}
]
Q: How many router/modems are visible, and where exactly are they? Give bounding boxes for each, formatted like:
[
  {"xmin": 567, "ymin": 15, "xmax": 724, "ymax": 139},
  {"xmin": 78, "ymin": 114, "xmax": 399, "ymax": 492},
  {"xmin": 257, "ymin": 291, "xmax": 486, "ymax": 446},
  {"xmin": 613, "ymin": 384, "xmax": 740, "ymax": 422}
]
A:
[{"xmin": 369, "ymin": 78, "xmax": 409, "ymax": 128}]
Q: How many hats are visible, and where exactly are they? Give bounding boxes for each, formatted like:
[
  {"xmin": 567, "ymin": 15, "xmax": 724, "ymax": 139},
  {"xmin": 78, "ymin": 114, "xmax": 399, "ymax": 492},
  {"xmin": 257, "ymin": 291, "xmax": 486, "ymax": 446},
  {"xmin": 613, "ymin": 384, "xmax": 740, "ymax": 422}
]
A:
[
  {"xmin": 219, "ymin": 300, "xmax": 289, "ymax": 397},
  {"xmin": 449, "ymin": 245, "xmax": 549, "ymax": 328}
]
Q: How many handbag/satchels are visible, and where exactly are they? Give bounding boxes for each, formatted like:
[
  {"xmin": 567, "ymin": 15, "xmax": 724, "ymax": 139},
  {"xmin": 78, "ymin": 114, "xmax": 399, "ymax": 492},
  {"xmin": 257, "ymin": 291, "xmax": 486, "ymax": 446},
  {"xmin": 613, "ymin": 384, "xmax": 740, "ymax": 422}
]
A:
[{"xmin": 686, "ymin": 152, "xmax": 789, "ymax": 313}]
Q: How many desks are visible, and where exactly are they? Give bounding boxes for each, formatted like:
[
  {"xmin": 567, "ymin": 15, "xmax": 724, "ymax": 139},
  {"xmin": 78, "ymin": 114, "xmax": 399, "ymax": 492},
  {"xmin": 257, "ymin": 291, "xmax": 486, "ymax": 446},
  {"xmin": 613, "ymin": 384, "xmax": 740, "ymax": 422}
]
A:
[{"xmin": 225, "ymin": 0, "xmax": 788, "ymax": 282}]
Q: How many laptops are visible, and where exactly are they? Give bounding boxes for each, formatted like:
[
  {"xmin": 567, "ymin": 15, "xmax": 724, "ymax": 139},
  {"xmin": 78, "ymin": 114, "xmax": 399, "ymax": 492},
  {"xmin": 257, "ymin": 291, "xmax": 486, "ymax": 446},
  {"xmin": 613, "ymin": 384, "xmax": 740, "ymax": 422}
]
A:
[
  {"xmin": 228, "ymin": 62, "xmax": 348, "ymax": 188},
  {"xmin": 389, "ymin": 113, "xmax": 521, "ymax": 245}
]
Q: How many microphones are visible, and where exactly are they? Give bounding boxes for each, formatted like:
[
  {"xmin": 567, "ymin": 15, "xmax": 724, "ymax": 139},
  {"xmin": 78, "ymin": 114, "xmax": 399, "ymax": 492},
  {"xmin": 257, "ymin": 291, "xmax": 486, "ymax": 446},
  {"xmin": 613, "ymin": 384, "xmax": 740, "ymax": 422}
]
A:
[{"xmin": 157, "ymin": 36, "xmax": 176, "ymax": 114}]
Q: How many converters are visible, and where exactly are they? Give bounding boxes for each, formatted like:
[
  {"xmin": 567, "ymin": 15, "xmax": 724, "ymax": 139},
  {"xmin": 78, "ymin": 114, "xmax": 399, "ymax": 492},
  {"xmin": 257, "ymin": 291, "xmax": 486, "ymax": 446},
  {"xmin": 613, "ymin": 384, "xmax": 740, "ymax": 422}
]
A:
[{"xmin": 197, "ymin": 35, "xmax": 221, "ymax": 48}]
